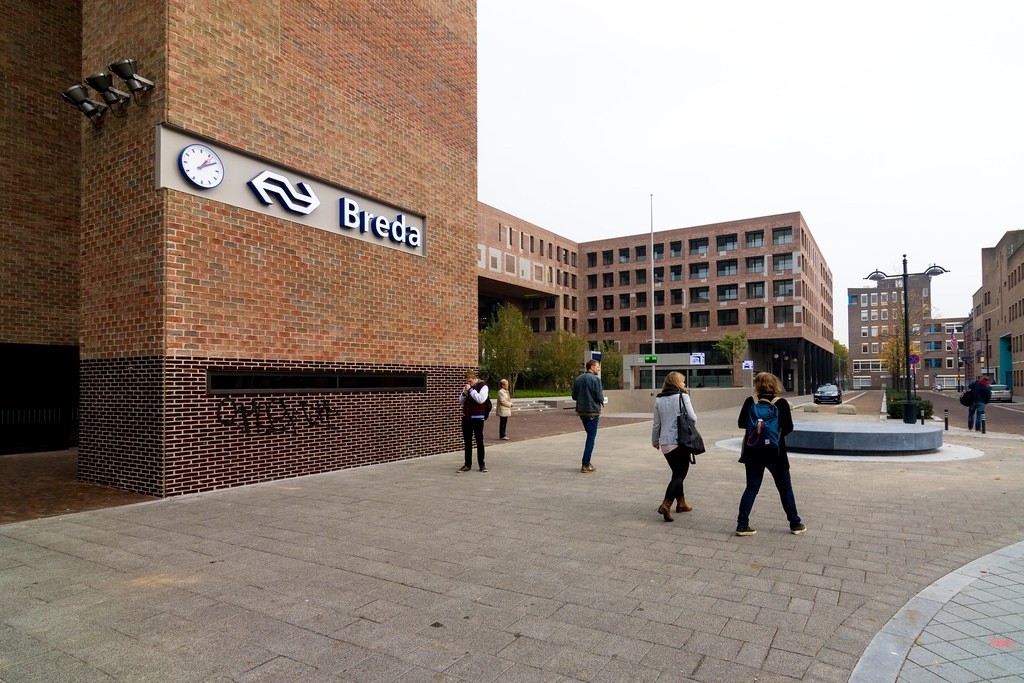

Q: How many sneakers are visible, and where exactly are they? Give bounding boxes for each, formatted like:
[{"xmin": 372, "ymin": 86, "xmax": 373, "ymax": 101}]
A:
[
  {"xmin": 791, "ymin": 523, "xmax": 806, "ymax": 535},
  {"xmin": 735, "ymin": 526, "xmax": 757, "ymax": 536}
]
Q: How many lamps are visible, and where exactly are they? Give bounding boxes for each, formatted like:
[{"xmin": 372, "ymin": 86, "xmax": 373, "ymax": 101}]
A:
[
  {"xmin": 108, "ymin": 57, "xmax": 155, "ymax": 93},
  {"xmin": 85, "ymin": 73, "xmax": 129, "ymax": 106},
  {"xmin": 62, "ymin": 82, "xmax": 103, "ymax": 121}
]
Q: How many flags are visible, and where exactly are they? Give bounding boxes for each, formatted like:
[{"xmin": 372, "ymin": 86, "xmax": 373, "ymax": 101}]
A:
[{"xmin": 950, "ymin": 332, "xmax": 956, "ymax": 349}]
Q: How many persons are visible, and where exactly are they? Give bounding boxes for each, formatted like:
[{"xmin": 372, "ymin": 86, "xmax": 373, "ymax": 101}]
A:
[
  {"xmin": 968, "ymin": 377, "xmax": 991, "ymax": 431},
  {"xmin": 496, "ymin": 379, "xmax": 513, "ymax": 440},
  {"xmin": 457, "ymin": 370, "xmax": 489, "ymax": 472},
  {"xmin": 572, "ymin": 359, "xmax": 604, "ymax": 473},
  {"xmin": 651, "ymin": 371, "xmax": 698, "ymax": 522},
  {"xmin": 735, "ymin": 371, "xmax": 807, "ymax": 536},
  {"xmin": 991, "ymin": 380, "xmax": 995, "ymax": 385}
]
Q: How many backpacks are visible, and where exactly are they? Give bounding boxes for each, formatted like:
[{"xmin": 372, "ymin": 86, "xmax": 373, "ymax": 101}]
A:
[
  {"xmin": 746, "ymin": 395, "xmax": 782, "ymax": 454},
  {"xmin": 960, "ymin": 383, "xmax": 979, "ymax": 407}
]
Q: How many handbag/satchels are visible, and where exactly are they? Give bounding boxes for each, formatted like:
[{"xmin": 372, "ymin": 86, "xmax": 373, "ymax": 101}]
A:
[{"xmin": 677, "ymin": 393, "xmax": 705, "ymax": 455}]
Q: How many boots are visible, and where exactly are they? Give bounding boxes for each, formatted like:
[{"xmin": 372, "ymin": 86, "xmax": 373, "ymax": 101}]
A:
[
  {"xmin": 676, "ymin": 496, "xmax": 692, "ymax": 513},
  {"xmin": 658, "ymin": 499, "xmax": 673, "ymax": 521}
]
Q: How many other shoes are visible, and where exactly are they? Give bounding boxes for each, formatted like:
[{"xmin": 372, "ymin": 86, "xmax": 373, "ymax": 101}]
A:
[
  {"xmin": 457, "ymin": 466, "xmax": 470, "ymax": 472},
  {"xmin": 501, "ymin": 436, "xmax": 510, "ymax": 440},
  {"xmin": 582, "ymin": 464, "xmax": 596, "ymax": 473},
  {"xmin": 480, "ymin": 466, "xmax": 487, "ymax": 472}
]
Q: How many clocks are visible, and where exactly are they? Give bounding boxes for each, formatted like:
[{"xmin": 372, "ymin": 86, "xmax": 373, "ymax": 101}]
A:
[{"xmin": 177, "ymin": 143, "xmax": 224, "ymax": 188}]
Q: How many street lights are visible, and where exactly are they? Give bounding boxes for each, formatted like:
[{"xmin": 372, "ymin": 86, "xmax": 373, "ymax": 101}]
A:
[
  {"xmin": 862, "ymin": 253, "xmax": 951, "ymax": 424},
  {"xmin": 976, "ymin": 334, "xmax": 990, "ymax": 376}
]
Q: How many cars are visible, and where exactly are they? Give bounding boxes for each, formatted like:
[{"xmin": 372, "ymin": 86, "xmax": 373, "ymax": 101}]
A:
[
  {"xmin": 990, "ymin": 385, "xmax": 1013, "ymax": 403},
  {"xmin": 814, "ymin": 382, "xmax": 842, "ymax": 404}
]
